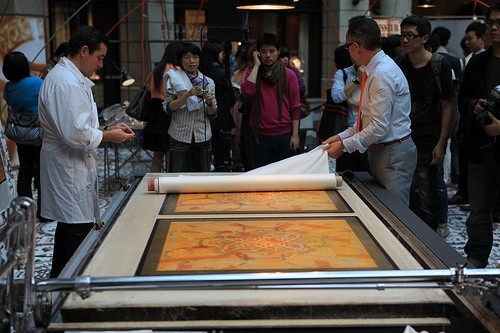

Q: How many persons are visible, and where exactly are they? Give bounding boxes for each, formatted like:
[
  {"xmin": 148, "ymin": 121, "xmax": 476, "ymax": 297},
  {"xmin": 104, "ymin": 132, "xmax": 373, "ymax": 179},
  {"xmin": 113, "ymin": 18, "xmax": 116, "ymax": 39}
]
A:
[
  {"xmin": 142, "ymin": 40, "xmax": 184, "ymax": 173},
  {"xmin": 240, "ymin": 33, "xmax": 303, "ymax": 172},
  {"xmin": 277, "ymin": 46, "xmax": 306, "ymax": 99},
  {"xmin": 38, "ymin": 42, "xmax": 70, "ymax": 80},
  {"xmin": 394, "ymin": 15, "xmax": 454, "ymax": 239},
  {"xmin": 331, "ymin": 57, "xmax": 372, "ymax": 174},
  {"xmin": 223, "ymin": 37, "xmax": 259, "ymax": 172},
  {"xmin": 380, "ymin": 14, "xmax": 495, "ymax": 211},
  {"xmin": 322, "ymin": 18, "xmax": 417, "ymax": 209},
  {"xmin": 198, "ymin": 40, "xmax": 238, "ymax": 171},
  {"xmin": 2, "ymin": 51, "xmax": 55, "ymax": 223},
  {"xmin": 38, "ymin": 25, "xmax": 135, "ymax": 278},
  {"xmin": 162, "ymin": 42, "xmax": 218, "ymax": 173},
  {"xmin": 457, "ymin": 0, "xmax": 500, "ymax": 268}
]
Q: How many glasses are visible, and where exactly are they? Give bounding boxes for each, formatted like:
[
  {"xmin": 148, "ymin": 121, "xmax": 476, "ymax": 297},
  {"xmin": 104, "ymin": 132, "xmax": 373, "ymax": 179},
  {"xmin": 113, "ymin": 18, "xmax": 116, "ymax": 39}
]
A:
[
  {"xmin": 343, "ymin": 41, "xmax": 360, "ymax": 49},
  {"xmin": 400, "ymin": 32, "xmax": 422, "ymax": 39},
  {"xmin": 486, "ymin": 20, "xmax": 500, "ymax": 27}
]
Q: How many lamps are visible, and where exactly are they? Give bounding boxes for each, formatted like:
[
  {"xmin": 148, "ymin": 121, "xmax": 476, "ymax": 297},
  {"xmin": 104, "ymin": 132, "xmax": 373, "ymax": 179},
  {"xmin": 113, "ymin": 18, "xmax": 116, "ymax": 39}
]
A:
[
  {"xmin": 416, "ymin": 1, "xmax": 437, "ymax": 9},
  {"xmin": 109, "ymin": 58, "xmax": 136, "ymax": 86},
  {"xmin": 235, "ymin": 0, "xmax": 296, "ymax": 11}
]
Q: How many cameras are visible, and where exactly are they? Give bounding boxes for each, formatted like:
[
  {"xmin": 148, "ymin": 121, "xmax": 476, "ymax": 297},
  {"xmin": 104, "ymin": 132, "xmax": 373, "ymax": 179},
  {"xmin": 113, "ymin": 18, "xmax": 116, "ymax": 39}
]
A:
[{"xmin": 475, "ymin": 101, "xmax": 500, "ymax": 129}]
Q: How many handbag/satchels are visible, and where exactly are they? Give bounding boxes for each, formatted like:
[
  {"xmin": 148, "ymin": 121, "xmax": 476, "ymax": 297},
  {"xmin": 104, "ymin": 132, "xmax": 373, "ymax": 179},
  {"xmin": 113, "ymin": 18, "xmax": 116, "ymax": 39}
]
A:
[
  {"xmin": 318, "ymin": 68, "xmax": 348, "ymax": 140},
  {"xmin": 300, "ymin": 96, "xmax": 310, "ymax": 119},
  {"xmin": 3, "ymin": 81, "xmax": 42, "ymax": 146},
  {"xmin": 125, "ymin": 66, "xmax": 170, "ymax": 129}
]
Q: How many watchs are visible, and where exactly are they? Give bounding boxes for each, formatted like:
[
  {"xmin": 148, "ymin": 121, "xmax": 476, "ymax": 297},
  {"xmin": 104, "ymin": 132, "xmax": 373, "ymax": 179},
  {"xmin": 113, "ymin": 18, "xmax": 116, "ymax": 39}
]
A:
[
  {"xmin": 205, "ymin": 101, "xmax": 213, "ymax": 106},
  {"xmin": 341, "ymin": 140, "xmax": 348, "ymax": 153},
  {"xmin": 352, "ymin": 78, "xmax": 360, "ymax": 86}
]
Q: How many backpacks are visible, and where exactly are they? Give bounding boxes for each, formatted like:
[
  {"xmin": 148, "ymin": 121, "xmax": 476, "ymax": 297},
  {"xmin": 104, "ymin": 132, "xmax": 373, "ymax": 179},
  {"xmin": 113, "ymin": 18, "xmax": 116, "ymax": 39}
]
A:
[{"xmin": 395, "ymin": 52, "xmax": 460, "ymax": 139}]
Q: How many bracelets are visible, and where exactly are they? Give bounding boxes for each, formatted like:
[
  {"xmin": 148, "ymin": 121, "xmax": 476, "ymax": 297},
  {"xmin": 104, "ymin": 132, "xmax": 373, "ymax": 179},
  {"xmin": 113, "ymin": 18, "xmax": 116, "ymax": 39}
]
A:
[{"xmin": 104, "ymin": 126, "xmax": 109, "ymax": 130}]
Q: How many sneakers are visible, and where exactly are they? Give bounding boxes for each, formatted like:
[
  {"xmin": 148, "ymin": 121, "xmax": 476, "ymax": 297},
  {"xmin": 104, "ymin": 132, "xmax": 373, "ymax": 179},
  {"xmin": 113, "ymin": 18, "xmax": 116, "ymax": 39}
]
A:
[
  {"xmin": 448, "ymin": 192, "xmax": 465, "ymax": 204},
  {"xmin": 437, "ymin": 225, "xmax": 450, "ymax": 238},
  {"xmin": 460, "ymin": 201, "xmax": 470, "ymax": 210}
]
